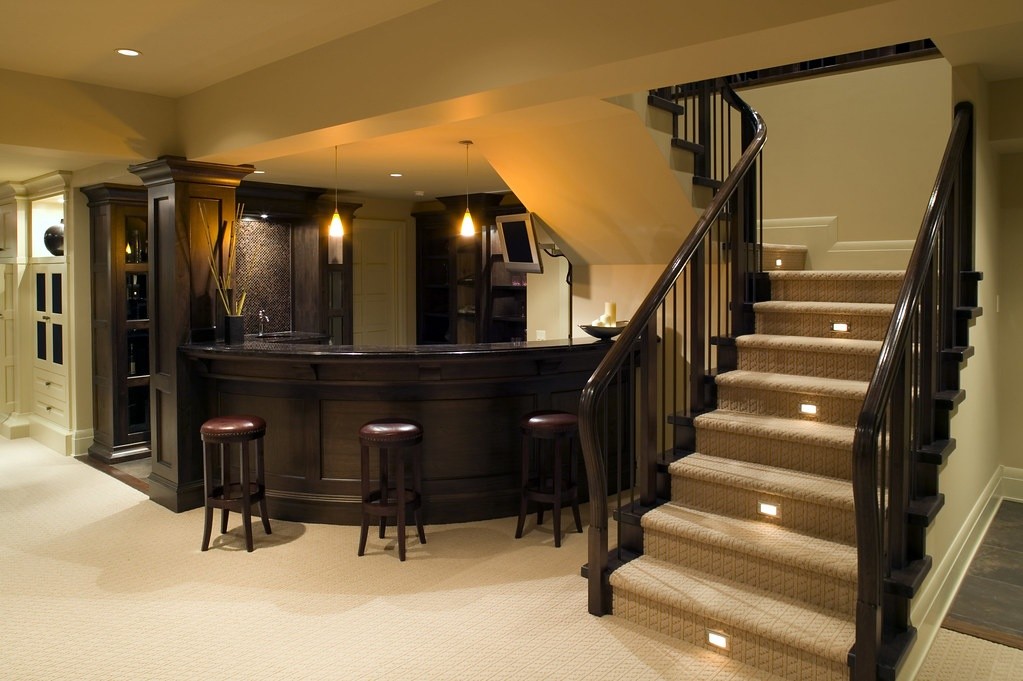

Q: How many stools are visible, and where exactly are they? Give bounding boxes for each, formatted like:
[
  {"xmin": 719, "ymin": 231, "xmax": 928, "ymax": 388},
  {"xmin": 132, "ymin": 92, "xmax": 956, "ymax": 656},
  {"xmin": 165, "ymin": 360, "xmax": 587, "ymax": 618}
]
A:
[
  {"xmin": 201, "ymin": 414, "xmax": 273, "ymax": 552},
  {"xmin": 514, "ymin": 410, "xmax": 583, "ymax": 548},
  {"xmin": 358, "ymin": 417, "xmax": 426, "ymax": 562}
]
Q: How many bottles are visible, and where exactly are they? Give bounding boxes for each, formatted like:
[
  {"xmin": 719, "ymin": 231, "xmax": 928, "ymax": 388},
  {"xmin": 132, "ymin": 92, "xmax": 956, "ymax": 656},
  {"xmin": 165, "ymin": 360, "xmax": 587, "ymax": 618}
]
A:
[{"xmin": 129, "ymin": 344, "xmax": 136, "ymax": 374}]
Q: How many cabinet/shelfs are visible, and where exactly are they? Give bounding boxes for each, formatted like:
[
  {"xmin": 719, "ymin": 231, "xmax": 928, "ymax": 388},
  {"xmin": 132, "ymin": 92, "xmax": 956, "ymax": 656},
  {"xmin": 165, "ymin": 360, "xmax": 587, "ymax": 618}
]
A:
[
  {"xmin": 0, "ymin": 170, "xmax": 95, "ymax": 456},
  {"xmin": 80, "ymin": 182, "xmax": 151, "ymax": 465},
  {"xmin": 413, "ymin": 205, "xmax": 529, "ymax": 347}
]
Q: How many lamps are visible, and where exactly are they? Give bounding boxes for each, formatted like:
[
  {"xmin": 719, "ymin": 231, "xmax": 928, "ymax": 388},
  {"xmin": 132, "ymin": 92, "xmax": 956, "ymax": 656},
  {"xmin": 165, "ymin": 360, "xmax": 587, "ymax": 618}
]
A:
[
  {"xmin": 329, "ymin": 145, "xmax": 345, "ymax": 238},
  {"xmin": 457, "ymin": 140, "xmax": 476, "ymax": 237}
]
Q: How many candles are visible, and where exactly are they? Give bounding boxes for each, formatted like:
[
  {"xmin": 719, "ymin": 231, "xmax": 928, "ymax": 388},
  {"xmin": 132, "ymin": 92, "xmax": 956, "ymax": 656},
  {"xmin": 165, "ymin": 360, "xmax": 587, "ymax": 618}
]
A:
[{"xmin": 592, "ymin": 302, "xmax": 617, "ymax": 327}]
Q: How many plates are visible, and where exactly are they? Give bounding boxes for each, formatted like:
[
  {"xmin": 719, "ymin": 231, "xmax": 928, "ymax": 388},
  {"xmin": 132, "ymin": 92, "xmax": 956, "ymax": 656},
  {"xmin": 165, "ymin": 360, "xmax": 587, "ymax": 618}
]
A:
[{"xmin": 578, "ymin": 320, "xmax": 627, "ymax": 340}]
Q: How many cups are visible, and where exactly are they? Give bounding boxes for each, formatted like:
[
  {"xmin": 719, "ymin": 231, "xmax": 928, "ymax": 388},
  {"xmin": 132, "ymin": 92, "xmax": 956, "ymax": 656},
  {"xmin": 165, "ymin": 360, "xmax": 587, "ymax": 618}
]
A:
[
  {"xmin": 521, "ymin": 275, "xmax": 528, "ymax": 286},
  {"xmin": 511, "ymin": 276, "xmax": 521, "ymax": 286}
]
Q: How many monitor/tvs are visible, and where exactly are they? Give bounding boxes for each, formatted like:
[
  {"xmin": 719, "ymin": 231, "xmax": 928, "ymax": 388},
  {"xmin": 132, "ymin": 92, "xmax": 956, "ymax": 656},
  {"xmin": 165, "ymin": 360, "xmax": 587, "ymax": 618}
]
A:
[{"xmin": 496, "ymin": 213, "xmax": 544, "ymax": 275}]
